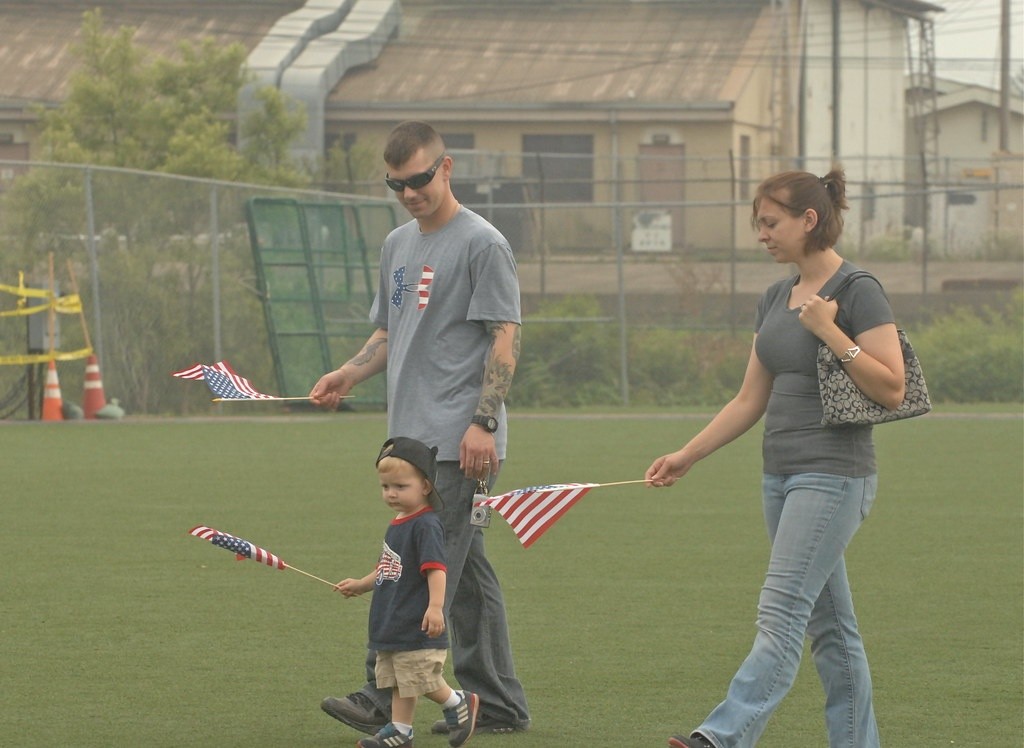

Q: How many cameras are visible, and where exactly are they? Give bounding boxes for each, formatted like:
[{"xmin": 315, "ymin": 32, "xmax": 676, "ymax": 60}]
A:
[{"xmin": 470, "ymin": 494, "xmax": 492, "ymax": 528}]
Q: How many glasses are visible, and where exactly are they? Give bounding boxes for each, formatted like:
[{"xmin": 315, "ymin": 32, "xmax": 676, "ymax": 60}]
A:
[{"xmin": 384, "ymin": 150, "xmax": 449, "ymax": 192}]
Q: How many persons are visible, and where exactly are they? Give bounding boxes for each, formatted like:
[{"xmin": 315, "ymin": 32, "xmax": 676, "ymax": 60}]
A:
[
  {"xmin": 332, "ymin": 437, "xmax": 480, "ymax": 748},
  {"xmin": 646, "ymin": 165, "xmax": 907, "ymax": 745},
  {"xmin": 321, "ymin": 121, "xmax": 528, "ymax": 739}
]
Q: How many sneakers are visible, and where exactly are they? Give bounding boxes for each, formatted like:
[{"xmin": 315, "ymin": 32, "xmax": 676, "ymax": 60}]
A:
[
  {"xmin": 442, "ymin": 689, "xmax": 480, "ymax": 748},
  {"xmin": 668, "ymin": 733, "xmax": 716, "ymax": 748},
  {"xmin": 320, "ymin": 692, "xmax": 391, "ymax": 736},
  {"xmin": 356, "ymin": 722, "xmax": 414, "ymax": 748}
]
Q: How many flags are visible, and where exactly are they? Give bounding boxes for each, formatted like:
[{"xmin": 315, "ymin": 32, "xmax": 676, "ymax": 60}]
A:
[
  {"xmin": 169, "ymin": 358, "xmax": 277, "ymax": 402},
  {"xmin": 186, "ymin": 524, "xmax": 287, "ymax": 572},
  {"xmin": 474, "ymin": 482, "xmax": 601, "ymax": 549}
]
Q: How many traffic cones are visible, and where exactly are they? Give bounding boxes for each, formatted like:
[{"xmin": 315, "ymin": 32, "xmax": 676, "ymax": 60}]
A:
[
  {"xmin": 82, "ymin": 355, "xmax": 107, "ymax": 419},
  {"xmin": 40, "ymin": 360, "xmax": 65, "ymax": 421}
]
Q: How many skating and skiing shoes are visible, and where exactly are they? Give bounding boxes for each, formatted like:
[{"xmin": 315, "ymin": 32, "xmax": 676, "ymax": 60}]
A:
[{"xmin": 431, "ymin": 710, "xmax": 531, "ymax": 734}]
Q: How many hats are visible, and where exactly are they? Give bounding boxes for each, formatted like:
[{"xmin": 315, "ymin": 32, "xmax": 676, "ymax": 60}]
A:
[{"xmin": 376, "ymin": 437, "xmax": 446, "ymax": 513}]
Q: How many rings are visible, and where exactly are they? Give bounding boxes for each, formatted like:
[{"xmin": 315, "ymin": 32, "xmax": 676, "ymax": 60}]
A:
[{"xmin": 483, "ymin": 460, "xmax": 490, "ymax": 464}]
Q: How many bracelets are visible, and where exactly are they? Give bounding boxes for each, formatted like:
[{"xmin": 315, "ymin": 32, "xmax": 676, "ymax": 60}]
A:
[{"xmin": 839, "ymin": 345, "xmax": 862, "ymax": 363}]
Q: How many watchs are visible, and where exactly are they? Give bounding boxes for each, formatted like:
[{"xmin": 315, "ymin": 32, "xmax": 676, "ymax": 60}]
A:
[{"xmin": 471, "ymin": 414, "xmax": 499, "ymax": 432}]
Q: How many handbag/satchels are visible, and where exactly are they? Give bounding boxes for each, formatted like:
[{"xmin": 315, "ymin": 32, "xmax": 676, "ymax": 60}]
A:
[{"xmin": 813, "ymin": 270, "xmax": 934, "ymax": 428}]
